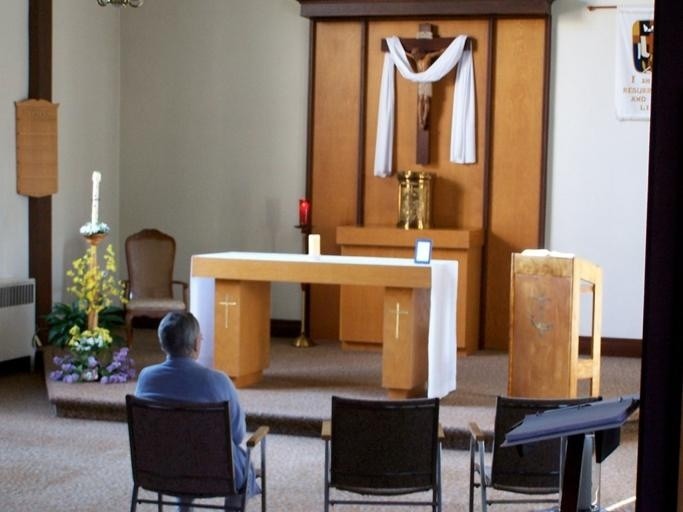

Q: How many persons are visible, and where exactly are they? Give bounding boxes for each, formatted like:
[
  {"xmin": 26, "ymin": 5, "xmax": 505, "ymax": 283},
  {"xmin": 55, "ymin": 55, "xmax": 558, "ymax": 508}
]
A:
[
  {"xmin": 130, "ymin": 310, "xmax": 262, "ymax": 512},
  {"xmin": 404, "ymin": 47, "xmax": 445, "ymax": 130}
]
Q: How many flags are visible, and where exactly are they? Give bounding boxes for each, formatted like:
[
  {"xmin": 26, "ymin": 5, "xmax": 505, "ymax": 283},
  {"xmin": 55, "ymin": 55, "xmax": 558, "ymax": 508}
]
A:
[{"xmin": 612, "ymin": 4, "xmax": 654, "ymax": 122}]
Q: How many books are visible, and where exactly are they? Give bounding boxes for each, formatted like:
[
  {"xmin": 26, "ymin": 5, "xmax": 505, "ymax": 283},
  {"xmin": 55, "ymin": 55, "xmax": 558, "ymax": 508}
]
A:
[{"xmin": 504, "ymin": 395, "xmax": 637, "ymax": 442}]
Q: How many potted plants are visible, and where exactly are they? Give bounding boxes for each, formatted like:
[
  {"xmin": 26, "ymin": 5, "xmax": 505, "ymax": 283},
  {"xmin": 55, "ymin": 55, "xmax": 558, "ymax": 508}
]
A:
[{"xmin": 42, "ymin": 297, "xmax": 127, "ymax": 375}]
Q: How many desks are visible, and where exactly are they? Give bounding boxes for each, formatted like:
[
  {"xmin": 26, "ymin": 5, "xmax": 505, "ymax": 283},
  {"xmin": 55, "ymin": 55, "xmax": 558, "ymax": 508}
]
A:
[
  {"xmin": 334, "ymin": 223, "xmax": 483, "ymax": 359},
  {"xmin": 191, "ymin": 252, "xmax": 459, "ymax": 402}
]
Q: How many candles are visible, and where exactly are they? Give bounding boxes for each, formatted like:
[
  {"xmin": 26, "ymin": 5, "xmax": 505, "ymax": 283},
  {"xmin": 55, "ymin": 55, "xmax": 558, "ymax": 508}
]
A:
[
  {"xmin": 90, "ymin": 180, "xmax": 99, "ymax": 230},
  {"xmin": 298, "ymin": 203, "xmax": 309, "ymax": 226}
]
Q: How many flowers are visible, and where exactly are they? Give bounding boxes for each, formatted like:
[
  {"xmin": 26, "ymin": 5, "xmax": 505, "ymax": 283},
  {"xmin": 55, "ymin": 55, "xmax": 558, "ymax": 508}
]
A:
[{"xmin": 49, "ymin": 243, "xmax": 137, "ymax": 388}]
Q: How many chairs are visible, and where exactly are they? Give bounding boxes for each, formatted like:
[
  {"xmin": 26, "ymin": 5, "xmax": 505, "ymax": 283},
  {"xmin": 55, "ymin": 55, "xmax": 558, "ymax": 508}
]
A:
[
  {"xmin": 123, "ymin": 226, "xmax": 190, "ymax": 350},
  {"xmin": 318, "ymin": 393, "xmax": 613, "ymax": 512},
  {"xmin": 124, "ymin": 391, "xmax": 271, "ymax": 512}
]
